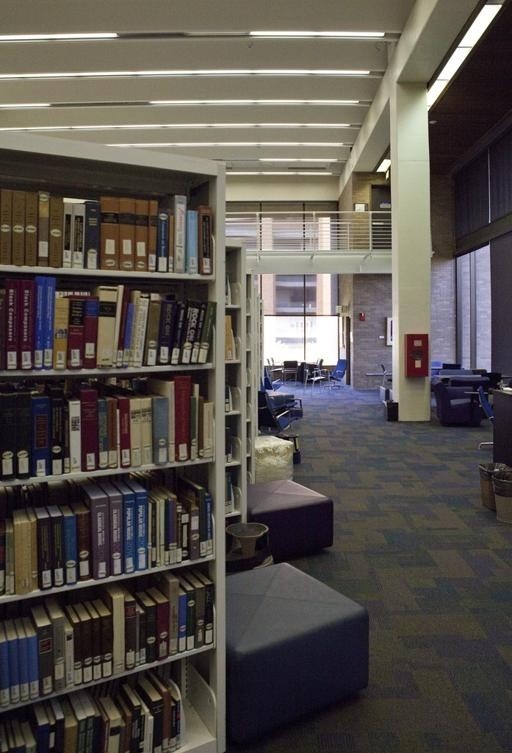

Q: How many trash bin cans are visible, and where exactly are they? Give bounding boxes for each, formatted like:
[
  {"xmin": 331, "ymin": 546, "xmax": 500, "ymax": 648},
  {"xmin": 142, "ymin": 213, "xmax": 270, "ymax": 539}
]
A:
[
  {"xmin": 479, "ymin": 462, "xmax": 508, "ymax": 511},
  {"xmin": 492, "ymin": 470, "xmax": 512, "ymax": 524},
  {"xmin": 383, "ymin": 401, "xmax": 398, "ymax": 421}
]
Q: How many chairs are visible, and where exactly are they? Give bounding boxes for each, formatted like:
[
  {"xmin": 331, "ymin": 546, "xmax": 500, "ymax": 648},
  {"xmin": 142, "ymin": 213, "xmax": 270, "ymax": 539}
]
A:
[
  {"xmin": 258, "ymin": 390, "xmax": 303, "ymax": 463},
  {"xmin": 431, "ymin": 360, "xmax": 501, "ymax": 428},
  {"xmin": 264, "ymin": 359, "xmax": 348, "ymax": 392},
  {"xmin": 475, "ymin": 386, "xmax": 494, "ymax": 449}
]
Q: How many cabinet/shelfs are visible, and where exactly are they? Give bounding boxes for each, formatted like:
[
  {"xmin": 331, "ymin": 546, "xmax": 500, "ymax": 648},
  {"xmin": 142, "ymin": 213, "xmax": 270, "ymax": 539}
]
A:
[
  {"xmin": 226, "ymin": 237, "xmax": 262, "ymax": 552},
  {"xmin": 0, "ymin": 130, "xmax": 226, "ymax": 752}
]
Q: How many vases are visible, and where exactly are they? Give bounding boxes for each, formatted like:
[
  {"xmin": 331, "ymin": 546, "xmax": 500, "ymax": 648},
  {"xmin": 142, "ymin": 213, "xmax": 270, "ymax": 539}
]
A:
[{"xmin": 226, "ymin": 521, "xmax": 269, "ymax": 565}]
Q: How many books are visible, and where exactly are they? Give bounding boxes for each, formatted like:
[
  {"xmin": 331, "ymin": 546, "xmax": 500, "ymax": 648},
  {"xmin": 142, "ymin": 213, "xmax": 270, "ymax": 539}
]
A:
[
  {"xmin": 0, "ymin": 371, "xmax": 214, "ymax": 479},
  {"xmin": 0, "ymin": 664, "xmax": 180, "ymax": 753},
  {"xmin": 1, "ymin": 476, "xmax": 213, "ymax": 594},
  {"xmin": 0, "ymin": 187, "xmax": 212, "ymax": 275},
  {"xmin": 0, "ymin": 568, "xmax": 214, "ymax": 707},
  {"xmin": 0, "ymin": 277, "xmax": 218, "ymax": 370},
  {"xmin": 225, "ymin": 274, "xmax": 235, "ymax": 504}
]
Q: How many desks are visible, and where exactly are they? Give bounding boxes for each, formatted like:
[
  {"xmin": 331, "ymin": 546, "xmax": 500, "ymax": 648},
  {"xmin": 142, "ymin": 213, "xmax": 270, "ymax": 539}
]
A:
[{"xmin": 492, "ymin": 390, "xmax": 511, "ymax": 466}]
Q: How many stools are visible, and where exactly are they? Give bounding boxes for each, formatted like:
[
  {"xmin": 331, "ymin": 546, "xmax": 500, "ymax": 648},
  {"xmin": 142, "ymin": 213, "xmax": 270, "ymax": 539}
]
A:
[
  {"xmin": 225, "ymin": 561, "xmax": 370, "ymax": 744},
  {"xmin": 246, "ymin": 479, "xmax": 333, "ymax": 561},
  {"xmin": 254, "ymin": 436, "xmax": 294, "ymax": 483}
]
